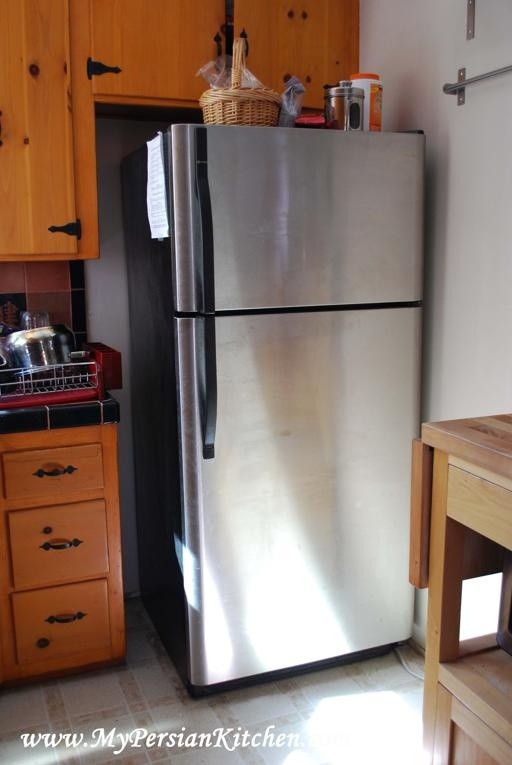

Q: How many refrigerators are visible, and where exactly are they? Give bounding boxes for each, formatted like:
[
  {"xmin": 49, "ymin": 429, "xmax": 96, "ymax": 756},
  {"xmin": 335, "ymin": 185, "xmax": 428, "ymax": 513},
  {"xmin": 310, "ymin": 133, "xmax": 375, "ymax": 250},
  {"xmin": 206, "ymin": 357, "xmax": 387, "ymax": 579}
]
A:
[{"xmin": 115, "ymin": 123, "xmax": 421, "ymax": 705}]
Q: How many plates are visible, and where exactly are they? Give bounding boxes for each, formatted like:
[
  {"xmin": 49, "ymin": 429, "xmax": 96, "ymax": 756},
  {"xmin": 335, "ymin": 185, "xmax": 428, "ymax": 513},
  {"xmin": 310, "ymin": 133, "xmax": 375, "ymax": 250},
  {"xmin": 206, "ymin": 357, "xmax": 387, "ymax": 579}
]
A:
[{"xmin": 289, "ymin": 115, "xmax": 325, "ymax": 128}]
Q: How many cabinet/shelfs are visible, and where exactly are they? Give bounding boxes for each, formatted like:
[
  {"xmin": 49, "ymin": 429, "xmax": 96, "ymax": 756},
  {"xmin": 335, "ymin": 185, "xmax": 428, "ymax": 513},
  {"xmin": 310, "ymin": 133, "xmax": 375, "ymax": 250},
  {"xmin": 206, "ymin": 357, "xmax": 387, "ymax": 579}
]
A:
[
  {"xmin": 0, "ymin": 0, "xmax": 99, "ymax": 263},
  {"xmin": 90, "ymin": 0, "xmax": 361, "ymax": 115},
  {"xmin": 0, "ymin": 393, "xmax": 127, "ymax": 686},
  {"xmin": 406, "ymin": 408, "xmax": 511, "ymax": 763}
]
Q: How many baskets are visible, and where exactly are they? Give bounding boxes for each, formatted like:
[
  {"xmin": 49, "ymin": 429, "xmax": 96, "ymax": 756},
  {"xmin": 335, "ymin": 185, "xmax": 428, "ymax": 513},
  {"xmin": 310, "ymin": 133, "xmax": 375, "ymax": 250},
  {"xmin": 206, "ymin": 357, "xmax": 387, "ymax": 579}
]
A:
[
  {"xmin": 0, "ymin": 358, "xmax": 98, "ymax": 398},
  {"xmin": 200, "ymin": 38, "xmax": 282, "ymax": 127}
]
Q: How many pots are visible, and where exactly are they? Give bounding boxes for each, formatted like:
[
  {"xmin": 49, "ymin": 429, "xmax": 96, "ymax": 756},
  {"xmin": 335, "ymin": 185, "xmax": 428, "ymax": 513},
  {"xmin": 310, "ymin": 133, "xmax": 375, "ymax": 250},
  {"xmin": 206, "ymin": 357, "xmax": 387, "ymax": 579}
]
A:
[{"xmin": 4, "ymin": 323, "xmax": 91, "ymax": 377}]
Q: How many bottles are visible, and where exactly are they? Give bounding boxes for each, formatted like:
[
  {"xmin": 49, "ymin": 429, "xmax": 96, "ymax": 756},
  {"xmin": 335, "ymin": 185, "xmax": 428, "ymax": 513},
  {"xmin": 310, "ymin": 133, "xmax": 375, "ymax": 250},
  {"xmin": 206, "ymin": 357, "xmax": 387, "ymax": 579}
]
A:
[
  {"xmin": 350, "ymin": 72, "xmax": 384, "ymax": 131},
  {"xmin": 323, "ymin": 78, "xmax": 362, "ymax": 130}
]
